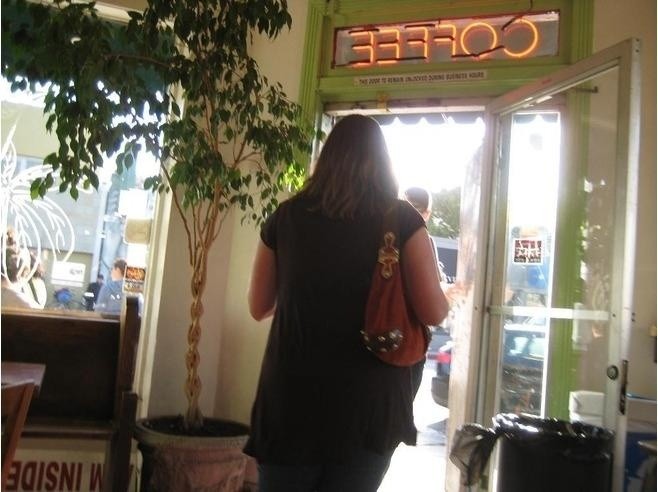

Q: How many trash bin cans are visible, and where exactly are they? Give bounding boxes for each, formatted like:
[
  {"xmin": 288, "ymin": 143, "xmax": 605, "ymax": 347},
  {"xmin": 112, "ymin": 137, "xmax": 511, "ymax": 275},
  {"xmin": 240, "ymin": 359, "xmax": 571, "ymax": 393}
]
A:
[{"xmin": 494, "ymin": 413, "xmax": 614, "ymax": 492}]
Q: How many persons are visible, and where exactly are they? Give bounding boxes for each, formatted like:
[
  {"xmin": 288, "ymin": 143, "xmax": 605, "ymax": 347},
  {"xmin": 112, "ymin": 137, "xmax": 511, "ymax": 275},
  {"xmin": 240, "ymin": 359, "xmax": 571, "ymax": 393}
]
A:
[
  {"xmin": 1, "ymin": 225, "xmax": 35, "ymax": 309},
  {"xmin": 22, "ymin": 251, "xmax": 48, "ymax": 309},
  {"xmin": 399, "ymin": 186, "xmax": 444, "ymax": 283},
  {"xmin": 86, "ymin": 274, "xmax": 105, "ymax": 311},
  {"xmin": 94, "ymin": 258, "xmax": 144, "ymax": 317},
  {"xmin": 242, "ymin": 114, "xmax": 451, "ymax": 492}
]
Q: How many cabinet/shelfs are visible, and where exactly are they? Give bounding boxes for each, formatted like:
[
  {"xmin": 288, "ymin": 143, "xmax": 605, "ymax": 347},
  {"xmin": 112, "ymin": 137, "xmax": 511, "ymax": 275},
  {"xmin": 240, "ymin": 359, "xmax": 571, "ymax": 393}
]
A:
[{"xmin": 0, "ymin": 290, "xmax": 145, "ymax": 492}]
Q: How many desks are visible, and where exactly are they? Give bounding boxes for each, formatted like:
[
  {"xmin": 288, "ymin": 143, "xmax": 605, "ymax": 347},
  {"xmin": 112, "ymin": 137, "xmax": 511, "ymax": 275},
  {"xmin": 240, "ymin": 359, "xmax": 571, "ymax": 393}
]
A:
[{"xmin": 1, "ymin": 362, "xmax": 52, "ymax": 397}]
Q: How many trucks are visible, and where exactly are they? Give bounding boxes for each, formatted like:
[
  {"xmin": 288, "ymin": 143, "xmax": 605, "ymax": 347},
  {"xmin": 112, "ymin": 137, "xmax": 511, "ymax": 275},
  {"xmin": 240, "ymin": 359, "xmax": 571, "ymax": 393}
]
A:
[
  {"xmin": 513, "ymin": 225, "xmax": 550, "ymax": 307},
  {"xmin": 428, "ymin": 233, "xmax": 461, "ymax": 327}
]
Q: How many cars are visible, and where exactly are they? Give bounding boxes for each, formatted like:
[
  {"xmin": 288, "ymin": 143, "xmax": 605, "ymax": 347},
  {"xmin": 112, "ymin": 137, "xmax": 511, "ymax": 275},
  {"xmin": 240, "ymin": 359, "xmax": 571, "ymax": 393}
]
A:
[{"xmin": 431, "ymin": 325, "xmax": 550, "ymax": 416}]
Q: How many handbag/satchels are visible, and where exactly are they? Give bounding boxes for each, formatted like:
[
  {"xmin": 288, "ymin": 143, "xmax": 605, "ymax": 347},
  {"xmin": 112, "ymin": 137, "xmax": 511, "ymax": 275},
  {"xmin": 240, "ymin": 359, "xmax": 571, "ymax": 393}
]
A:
[{"xmin": 360, "ymin": 246, "xmax": 431, "ymax": 368}]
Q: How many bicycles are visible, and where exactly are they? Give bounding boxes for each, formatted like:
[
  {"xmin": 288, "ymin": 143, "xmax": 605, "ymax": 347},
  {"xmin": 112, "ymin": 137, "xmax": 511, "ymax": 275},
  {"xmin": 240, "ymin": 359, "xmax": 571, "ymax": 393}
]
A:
[{"xmin": 49, "ymin": 290, "xmax": 95, "ymax": 313}]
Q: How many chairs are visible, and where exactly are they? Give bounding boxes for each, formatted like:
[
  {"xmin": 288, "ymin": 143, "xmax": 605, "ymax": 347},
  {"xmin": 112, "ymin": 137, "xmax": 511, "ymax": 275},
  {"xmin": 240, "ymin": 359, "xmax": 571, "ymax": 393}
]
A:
[{"xmin": 0, "ymin": 375, "xmax": 36, "ymax": 492}]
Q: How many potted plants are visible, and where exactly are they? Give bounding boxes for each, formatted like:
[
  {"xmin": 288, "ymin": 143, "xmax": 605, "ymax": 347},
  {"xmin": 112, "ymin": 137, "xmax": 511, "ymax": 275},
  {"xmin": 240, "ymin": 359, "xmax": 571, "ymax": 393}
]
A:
[{"xmin": 0, "ymin": 0, "xmax": 338, "ymax": 492}]
